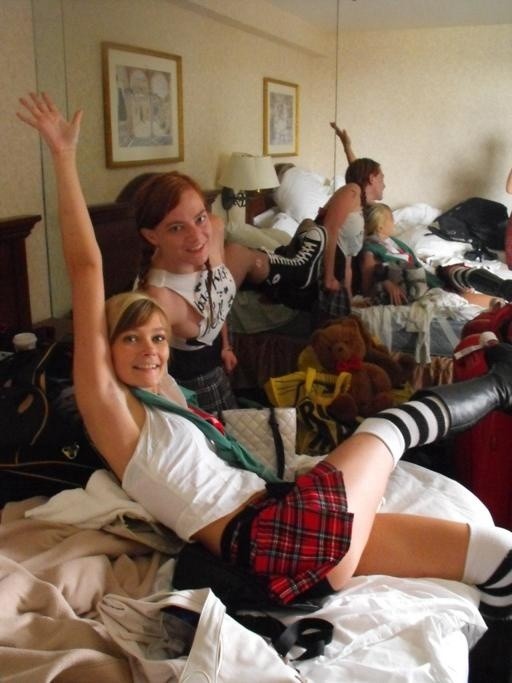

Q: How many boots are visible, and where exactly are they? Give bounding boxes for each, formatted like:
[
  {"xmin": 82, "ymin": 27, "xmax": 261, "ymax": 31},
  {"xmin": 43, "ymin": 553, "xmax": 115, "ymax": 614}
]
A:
[
  {"xmin": 408, "ymin": 339, "xmax": 512, "ymax": 449},
  {"xmin": 467, "ymin": 265, "xmax": 512, "ymax": 303},
  {"xmin": 254, "ymin": 224, "xmax": 327, "ymax": 292}
]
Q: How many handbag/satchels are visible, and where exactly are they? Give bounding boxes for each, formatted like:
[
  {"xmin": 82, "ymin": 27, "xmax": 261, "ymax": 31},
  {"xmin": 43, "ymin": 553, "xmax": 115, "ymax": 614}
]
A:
[{"xmin": 263, "ymin": 365, "xmax": 362, "ymax": 459}]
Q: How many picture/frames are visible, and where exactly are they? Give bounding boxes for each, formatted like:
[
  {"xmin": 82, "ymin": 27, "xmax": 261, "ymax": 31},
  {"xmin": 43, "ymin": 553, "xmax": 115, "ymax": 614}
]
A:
[
  {"xmin": 99, "ymin": 39, "xmax": 185, "ymax": 170},
  {"xmin": 262, "ymin": 76, "xmax": 302, "ymax": 159}
]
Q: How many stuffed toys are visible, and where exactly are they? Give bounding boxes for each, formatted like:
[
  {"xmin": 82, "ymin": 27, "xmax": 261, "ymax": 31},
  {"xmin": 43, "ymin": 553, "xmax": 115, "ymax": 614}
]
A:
[{"xmin": 312, "ymin": 309, "xmax": 509, "ymax": 419}]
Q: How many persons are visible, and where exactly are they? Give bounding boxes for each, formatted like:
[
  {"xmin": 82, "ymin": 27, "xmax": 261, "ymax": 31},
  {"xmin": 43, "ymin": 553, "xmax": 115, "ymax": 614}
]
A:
[
  {"xmin": 359, "ymin": 202, "xmax": 512, "ymax": 309},
  {"xmin": 116, "ymin": 171, "xmax": 329, "ymax": 411},
  {"xmin": 300, "ymin": 159, "xmax": 385, "ymax": 335},
  {"xmin": 16, "ymin": 85, "xmax": 510, "ymax": 616}
]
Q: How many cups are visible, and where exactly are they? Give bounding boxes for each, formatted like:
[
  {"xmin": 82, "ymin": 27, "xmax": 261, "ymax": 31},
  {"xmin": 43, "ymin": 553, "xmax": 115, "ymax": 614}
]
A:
[{"xmin": 12, "ymin": 332, "xmax": 37, "ymax": 353}]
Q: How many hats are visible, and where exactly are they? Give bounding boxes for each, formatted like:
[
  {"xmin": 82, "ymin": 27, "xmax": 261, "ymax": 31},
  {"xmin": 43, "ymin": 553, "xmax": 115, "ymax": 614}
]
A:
[{"xmin": 102, "ymin": 291, "xmax": 175, "ymax": 351}]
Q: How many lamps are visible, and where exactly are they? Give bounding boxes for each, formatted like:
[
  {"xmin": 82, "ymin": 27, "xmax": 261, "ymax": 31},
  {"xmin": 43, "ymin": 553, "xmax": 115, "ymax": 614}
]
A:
[{"xmin": 217, "ymin": 149, "xmax": 279, "ymax": 210}]
